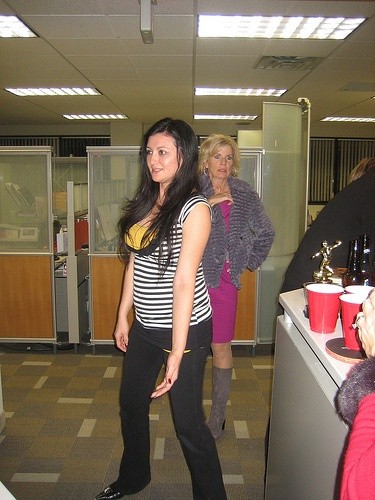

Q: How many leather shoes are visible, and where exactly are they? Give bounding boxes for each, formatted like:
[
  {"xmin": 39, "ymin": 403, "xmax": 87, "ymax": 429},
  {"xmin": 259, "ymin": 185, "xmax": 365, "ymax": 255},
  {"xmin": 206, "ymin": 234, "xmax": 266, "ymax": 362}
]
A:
[{"xmin": 95, "ymin": 481, "xmax": 124, "ymax": 500}]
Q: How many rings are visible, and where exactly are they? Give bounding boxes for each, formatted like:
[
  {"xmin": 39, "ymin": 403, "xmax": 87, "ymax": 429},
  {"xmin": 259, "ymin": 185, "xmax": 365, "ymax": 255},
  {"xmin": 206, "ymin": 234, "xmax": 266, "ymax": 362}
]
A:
[{"xmin": 351, "ymin": 315, "xmax": 364, "ymax": 330}]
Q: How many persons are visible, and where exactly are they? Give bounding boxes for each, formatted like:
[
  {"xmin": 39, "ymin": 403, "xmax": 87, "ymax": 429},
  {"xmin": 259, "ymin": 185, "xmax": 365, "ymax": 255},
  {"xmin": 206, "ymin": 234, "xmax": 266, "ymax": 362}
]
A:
[
  {"xmin": 95, "ymin": 118, "xmax": 228, "ymax": 500},
  {"xmin": 195, "ymin": 134, "xmax": 275, "ymax": 440},
  {"xmin": 336, "ymin": 289, "xmax": 375, "ymax": 500},
  {"xmin": 312, "ymin": 240, "xmax": 342, "ymax": 277},
  {"xmin": 276, "ymin": 155, "xmax": 375, "ymax": 314}
]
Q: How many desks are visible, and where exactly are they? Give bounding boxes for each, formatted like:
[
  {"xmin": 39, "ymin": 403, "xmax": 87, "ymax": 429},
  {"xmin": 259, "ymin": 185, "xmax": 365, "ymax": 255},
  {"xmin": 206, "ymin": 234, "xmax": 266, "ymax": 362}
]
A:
[
  {"xmin": 264, "ymin": 286, "xmax": 369, "ymax": 500},
  {"xmin": 54, "ymin": 213, "xmax": 90, "ymax": 353}
]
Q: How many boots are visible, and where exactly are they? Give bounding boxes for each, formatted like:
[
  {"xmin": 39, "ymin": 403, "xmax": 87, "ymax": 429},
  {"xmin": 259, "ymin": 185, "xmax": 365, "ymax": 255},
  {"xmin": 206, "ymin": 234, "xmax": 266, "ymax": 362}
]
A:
[{"xmin": 208, "ymin": 364, "xmax": 234, "ymax": 439}]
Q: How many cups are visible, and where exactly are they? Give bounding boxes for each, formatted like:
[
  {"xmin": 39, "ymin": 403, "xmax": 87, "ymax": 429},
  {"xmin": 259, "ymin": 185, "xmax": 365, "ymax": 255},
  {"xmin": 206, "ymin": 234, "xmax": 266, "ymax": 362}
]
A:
[
  {"xmin": 303, "ymin": 281, "xmax": 318, "ymax": 316},
  {"xmin": 345, "ymin": 285, "xmax": 375, "ymax": 295},
  {"xmin": 305, "ymin": 283, "xmax": 345, "ymax": 334},
  {"xmin": 338, "ymin": 293, "xmax": 365, "ymax": 351}
]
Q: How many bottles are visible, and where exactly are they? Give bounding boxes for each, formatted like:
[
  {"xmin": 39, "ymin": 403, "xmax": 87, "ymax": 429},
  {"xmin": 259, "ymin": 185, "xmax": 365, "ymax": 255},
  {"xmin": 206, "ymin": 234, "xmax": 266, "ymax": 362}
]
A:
[
  {"xmin": 342, "ymin": 239, "xmax": 364, "ymax": 288},
  {"xmin": 358, "ymin": 233, "xmax": 372, "ymax": 286}
]
